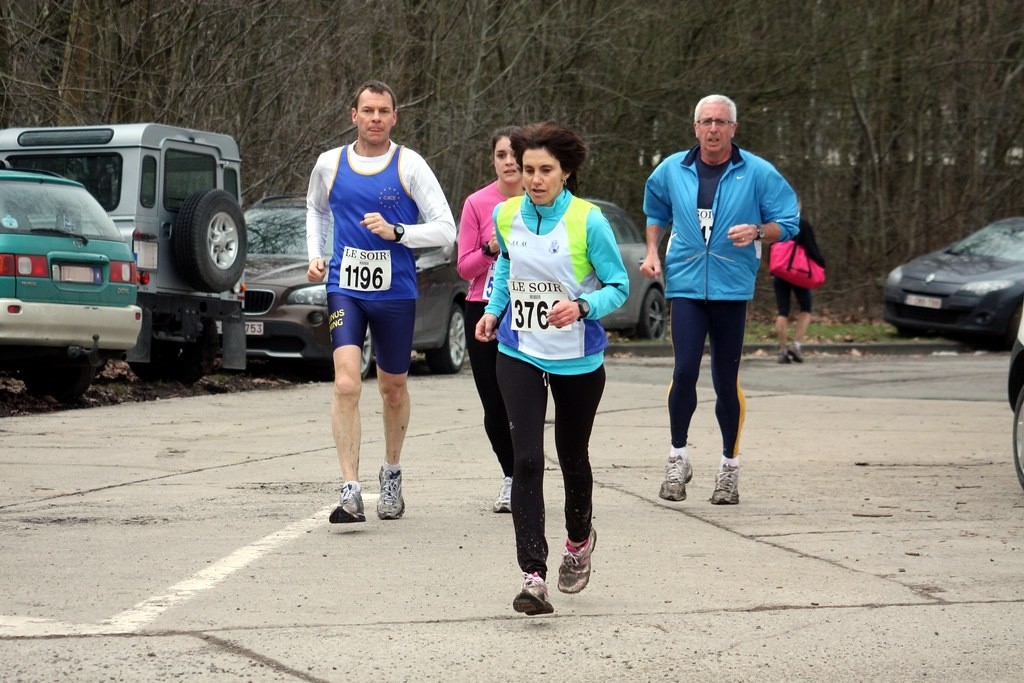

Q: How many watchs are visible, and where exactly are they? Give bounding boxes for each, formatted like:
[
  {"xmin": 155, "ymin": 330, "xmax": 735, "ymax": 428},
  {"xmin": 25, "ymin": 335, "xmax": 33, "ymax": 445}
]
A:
[
  {"xmin": 572, "ymin": 298, "xmax": 590, "ymax": 322},
  {"xmin": 753, "ymin": 224, "xmax": 765, "ymax": 242},
  {"xmin": 482, "ymin": 241, "xmax": 498, "ymax": 257},
  {"xmin": 392, "ymin": 223, "xmax": 404, "ymax": 243}
]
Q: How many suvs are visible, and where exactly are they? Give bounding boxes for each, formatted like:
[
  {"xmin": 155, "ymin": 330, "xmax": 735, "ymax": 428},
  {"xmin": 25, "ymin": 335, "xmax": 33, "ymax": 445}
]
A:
[
  {"xmin": 0, "ymin": 166, "xmax": 143, "ymax": 403},
  {"xmin": 216, "ymin": 195, "xmax": 471, "ymax": 381},
  {"xmin": 0, "ymin": 123, "xmax": 246, "ymax": 382}
]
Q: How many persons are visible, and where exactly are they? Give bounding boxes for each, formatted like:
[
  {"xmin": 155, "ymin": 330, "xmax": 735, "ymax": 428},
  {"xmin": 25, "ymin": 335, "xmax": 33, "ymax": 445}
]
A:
[
  {"xmin": 457, "ymin": 128, "xmax": 549, "ymax": 512},
  {"xmin": 767, "ymin": 197, "xmax": 825, "ymax": 364},
  {"xmin": 305, "ymin": 80, "xmax": 455, "ymax": 524},
  {"xmin": 637, "ymin": 95, "xmax": 801, "ymax": 503},
  {"xmin": 473, "ymin": 121, "xmax": 630, "ymax": 616}
]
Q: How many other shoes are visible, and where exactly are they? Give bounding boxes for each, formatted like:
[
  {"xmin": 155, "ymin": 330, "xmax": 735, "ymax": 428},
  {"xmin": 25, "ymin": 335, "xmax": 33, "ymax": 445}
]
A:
[
  {"xmin": 788, "ymin": 343, "xmax": 803, "ymax": 363},
  {"xmin": 777, "ymin": 351, "xmax": 792, "ymax": 363}
]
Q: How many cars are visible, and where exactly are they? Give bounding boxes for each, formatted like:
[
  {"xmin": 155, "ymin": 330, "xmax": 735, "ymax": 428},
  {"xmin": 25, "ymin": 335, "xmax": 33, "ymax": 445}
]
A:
[
  {"xmin": 881, "ymin": 216, "xmax": 1024, "ymax": 351},
  {"xmin": 584, "ymin": 199, "xmax": 667, "ymax": 342},
  {"xmin": 1007, "ymin": 306, "xmax": 1024, "ymax": 489}
]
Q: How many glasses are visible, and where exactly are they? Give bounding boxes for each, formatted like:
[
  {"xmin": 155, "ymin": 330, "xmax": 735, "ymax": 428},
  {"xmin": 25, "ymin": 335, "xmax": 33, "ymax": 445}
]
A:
[{"xmin": 698, "ymin": 118, "xmax": 734, "ymax": 127}]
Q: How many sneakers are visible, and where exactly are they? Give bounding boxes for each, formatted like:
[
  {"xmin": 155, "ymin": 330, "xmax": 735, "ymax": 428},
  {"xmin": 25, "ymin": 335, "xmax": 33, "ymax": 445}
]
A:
[
  {"xmin": 493, "ymin": 475, "xmax": 514, "ymax": 512},
  {"xmin": 712, "ymin": 463, "xmax": 740, "ymax": 503},
  {"xmin": 377, "ymin": 467, "xmax": 405, "ymax": 518},
  {"xmin": 513, "ymin": 572, "xmax": 553, "ymax": 615},
  {"xmin": 558, "ymin": 526, "xmax": 597, "ymax": 592},
  {"xmin": 328, "ymin": 482, "xmax": 366, "ymax": 523},
  {"xmin": 659, "ymin": 455, "xmax": 692, "ymax": 501}
]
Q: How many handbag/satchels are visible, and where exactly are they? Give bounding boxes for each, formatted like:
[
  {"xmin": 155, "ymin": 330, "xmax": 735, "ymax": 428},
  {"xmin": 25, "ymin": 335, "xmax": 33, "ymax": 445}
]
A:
[{"xmin": 769, "ymin": 238, "xmax": 825, "ymax": 288}]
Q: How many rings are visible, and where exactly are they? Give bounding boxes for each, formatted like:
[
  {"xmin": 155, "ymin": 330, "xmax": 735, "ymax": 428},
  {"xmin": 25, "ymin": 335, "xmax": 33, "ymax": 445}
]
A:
[{"xmin": 742, "ymin": 237, "xmax": 745, "ymax": 242}]
